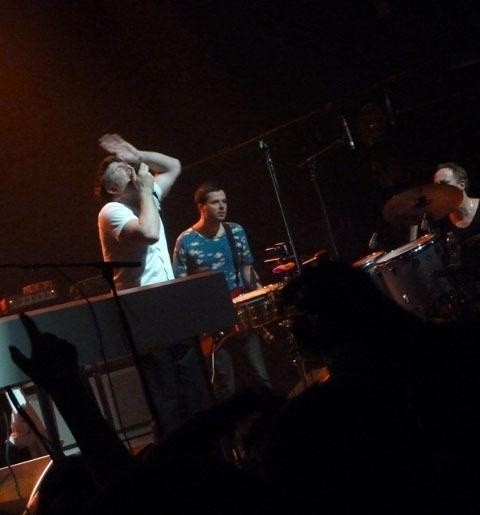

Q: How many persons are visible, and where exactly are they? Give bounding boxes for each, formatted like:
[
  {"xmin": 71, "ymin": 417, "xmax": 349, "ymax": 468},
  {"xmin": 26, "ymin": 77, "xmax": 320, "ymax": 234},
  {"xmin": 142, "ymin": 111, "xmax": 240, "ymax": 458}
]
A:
[
  {"xmin": 170, "ymin": 178, "xmax": 273, "ymax": 427},
  {"xmin": 92, "ymin": 130, "xmax": 216, "ymax": 435},
  {"xmin": 1, "ymin": 254, "xmax": 478, "ymax": 515},
  {"xmin": 432, "ymin": 159, "xmax": 480, "ymax": 268}
]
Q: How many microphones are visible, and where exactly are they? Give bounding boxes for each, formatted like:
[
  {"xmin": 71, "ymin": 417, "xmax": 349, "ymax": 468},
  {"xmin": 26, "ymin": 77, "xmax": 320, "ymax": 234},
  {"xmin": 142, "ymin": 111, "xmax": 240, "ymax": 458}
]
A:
[
  {"xmin": 134, "ymin": 167, "xmax": 159, "ymax": 202},
  {"xmin": 336, "ymin": 109, "xmax": 358, "ymax": 153}
]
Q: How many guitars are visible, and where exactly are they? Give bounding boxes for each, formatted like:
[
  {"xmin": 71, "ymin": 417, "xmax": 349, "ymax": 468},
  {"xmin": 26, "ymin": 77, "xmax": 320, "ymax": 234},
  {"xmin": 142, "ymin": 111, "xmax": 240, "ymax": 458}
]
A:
[{"xmin": 199, "ymin": 248, "xmax": 326, "ymax": 359}]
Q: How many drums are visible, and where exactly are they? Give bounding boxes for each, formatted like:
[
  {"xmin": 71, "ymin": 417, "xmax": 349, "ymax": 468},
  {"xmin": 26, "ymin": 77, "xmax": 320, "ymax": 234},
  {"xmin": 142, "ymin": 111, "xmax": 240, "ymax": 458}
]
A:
[
  {"xmin": 373, "ymin": 233, "xmax": 456, "ymax": 311},
  {"xmin": 353, "ymin": 251, "xmax": 392, "ymax": 299},
  {"xmin": 232, "ymin": 283, "xmax": 286, "ymax": 328}
]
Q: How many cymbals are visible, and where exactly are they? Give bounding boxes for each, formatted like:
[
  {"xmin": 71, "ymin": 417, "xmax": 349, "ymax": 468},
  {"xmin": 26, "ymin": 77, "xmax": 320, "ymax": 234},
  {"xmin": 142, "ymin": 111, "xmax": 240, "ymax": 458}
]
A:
[{"xmin": 383, "ymin": 184, "xmax": 463, "ymax": 225}]
